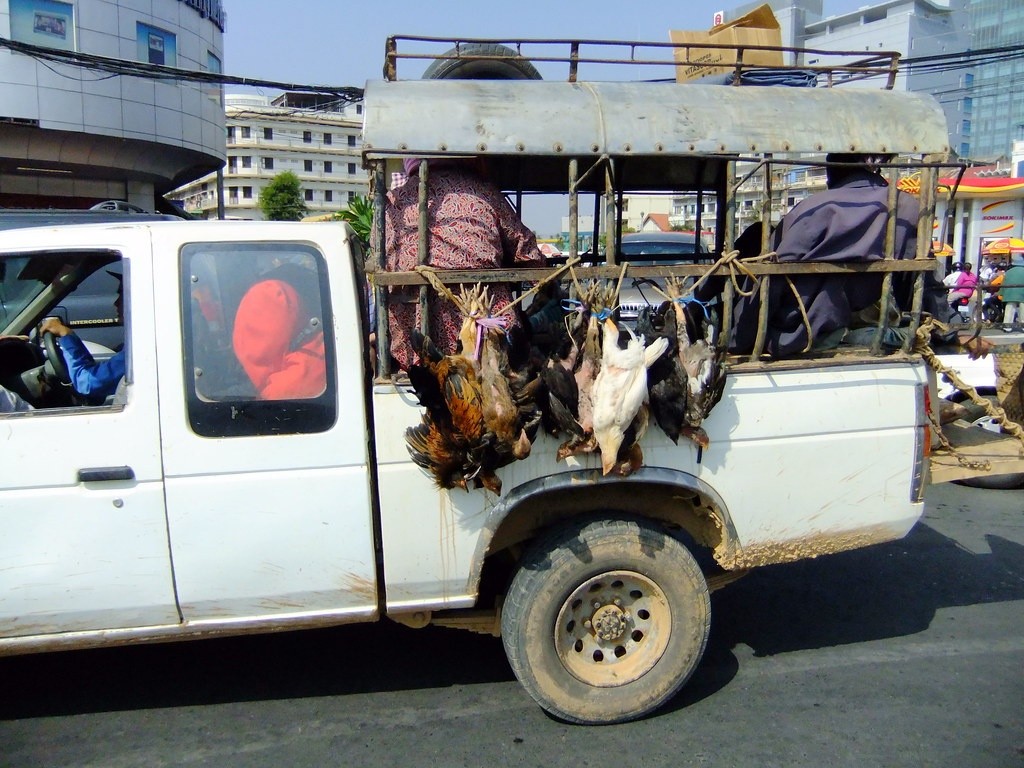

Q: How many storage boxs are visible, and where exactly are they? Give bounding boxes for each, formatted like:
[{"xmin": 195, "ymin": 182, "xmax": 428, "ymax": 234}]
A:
[{"xmin": 668, "ymin": 3, "xmax": 785, "ymax": 84}]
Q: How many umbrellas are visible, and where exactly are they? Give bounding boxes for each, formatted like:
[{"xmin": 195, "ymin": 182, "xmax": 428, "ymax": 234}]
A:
[
  {"xmin": 932, "ymin": 241, "xmax": 955, "ymax": 257},
  {"xmin": 981, "ymin": 237, "xmax": 1024, "ymax": 264},
  {"xmin": 536, "ymin": 243, "xmax": 560, "ymax": 257}
]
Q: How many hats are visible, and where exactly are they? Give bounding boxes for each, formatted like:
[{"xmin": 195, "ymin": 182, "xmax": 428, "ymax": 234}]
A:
[
  {"xmin": 106, "ymin": 270, "xmax": 123, "ymax": 282},
  {"xmin": 1011, "ymin": 256, "xmax": 1024, "ymax": 266}
]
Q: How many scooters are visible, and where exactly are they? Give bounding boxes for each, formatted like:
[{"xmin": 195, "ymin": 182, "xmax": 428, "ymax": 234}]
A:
[{"xmin": 952, "ymin": 298, "xmax": 970, "ymax": 323}]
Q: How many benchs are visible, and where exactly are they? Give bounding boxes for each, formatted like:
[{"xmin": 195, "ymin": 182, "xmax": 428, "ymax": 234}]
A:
[{"xmin": 843, "ymin": 324, "xmax": 908, "ymax": 348}]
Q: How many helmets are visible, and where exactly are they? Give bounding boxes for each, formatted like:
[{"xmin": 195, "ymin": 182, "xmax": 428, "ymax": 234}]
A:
[
  {"xmin": 997, "ymin": 262, "xmax": 1007, "ymax": 270},
  {"xmin": 952, "ymin": 262, "xmax": 964, "ymax": 271}
]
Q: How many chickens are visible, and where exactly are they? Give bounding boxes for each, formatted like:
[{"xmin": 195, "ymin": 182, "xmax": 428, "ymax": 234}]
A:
[
  {"xmin": 541, "ymin": 272, "xmax": 728, "ymax": 476},
  {"xmin": 404, "ymin": 280, "xmax": 542, "ymax": 497}
]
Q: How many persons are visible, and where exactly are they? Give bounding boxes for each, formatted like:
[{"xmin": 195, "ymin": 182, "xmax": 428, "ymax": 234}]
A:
[
  {"xmin": 368, "ymin": 156, "xmax": 546, "ymax": 375},
  {"xmin": 526, "ymin": 280, "xmax": 580, "ymax": 332},
  {"xmin": 701, "ymin": 153, "xmax": 961, "ymax": 361},
  {"xmin": 37, "ymin": 268, "xmax": 126, "ymax": 400},
  {"xmin": 208, "ymin": 264, "xmax": 321, "ymax": 401},
  {"xmin": 979, "ymin": 261, "xmax": 1014, "ymax": 324},
  {"xmin": 34, "ymin": 16, "xmax": 65, "ymax": 36},
  {"xmin": 942, "ymin": 261, "xmax": 979, "ymax": 305}
]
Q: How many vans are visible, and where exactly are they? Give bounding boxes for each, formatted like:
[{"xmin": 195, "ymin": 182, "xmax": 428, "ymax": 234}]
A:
[{"xmin": 596, "ymin": 232, "xmax": 716, "ymax": 321}]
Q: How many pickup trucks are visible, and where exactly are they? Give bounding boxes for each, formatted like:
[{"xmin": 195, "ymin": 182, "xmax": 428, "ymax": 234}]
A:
[{"xmin": 0, "ymin": 35, "xmax": 1024, "ymax": 726}]
